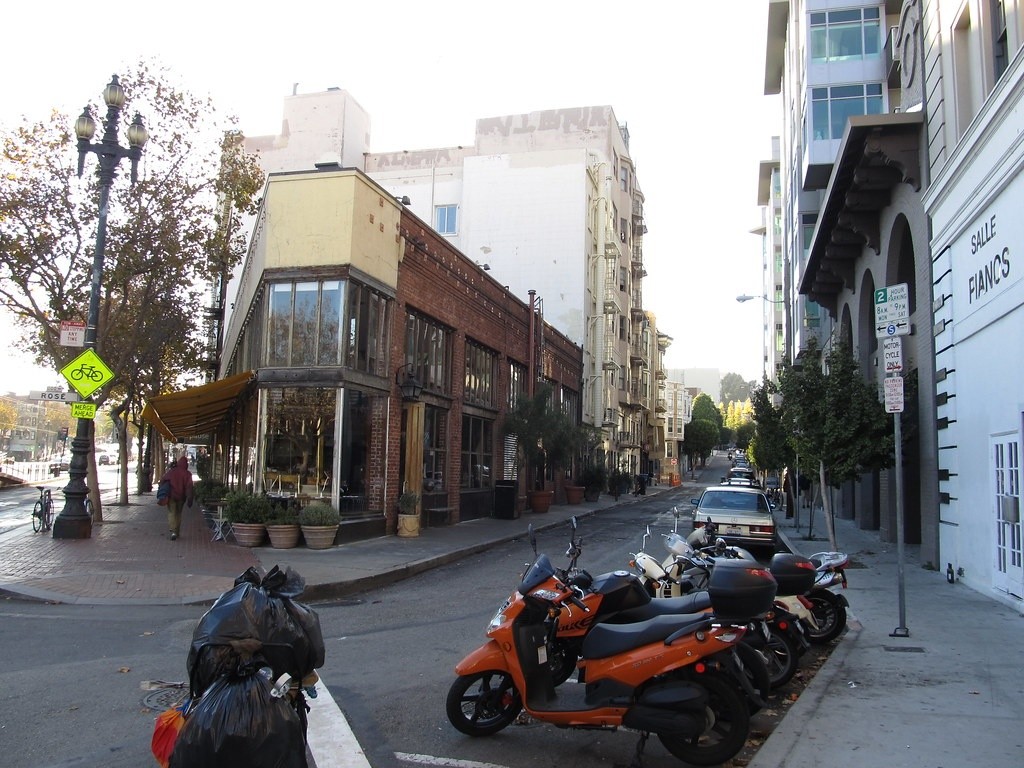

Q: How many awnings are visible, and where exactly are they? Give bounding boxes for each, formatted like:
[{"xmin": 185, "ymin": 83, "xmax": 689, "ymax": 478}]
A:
[{"xmin": 140, "ymin": 369, "xmax": 255, "ymax": 446}]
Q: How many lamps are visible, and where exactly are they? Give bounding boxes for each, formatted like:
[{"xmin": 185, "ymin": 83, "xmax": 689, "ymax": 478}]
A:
[
  {"xmin": 396, "ymin": 363, "xmax": 423, "ymax": 401},
  {"xmin": 396, "ymin": 196, "xmax": 411, "ymax": 206},
  {"xmin": 479, "ymin": 264, "xmax": 490, "ymax": 270}
]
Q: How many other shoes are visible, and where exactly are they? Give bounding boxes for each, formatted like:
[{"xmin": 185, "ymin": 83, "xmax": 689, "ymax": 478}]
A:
[{"xmin": 170, "ymin": 533, "xmax": 177, "ymax": 541}]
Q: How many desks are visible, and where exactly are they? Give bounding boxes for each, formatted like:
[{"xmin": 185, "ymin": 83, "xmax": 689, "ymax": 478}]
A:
[
  {"xmin": 301, "ymin": 484, "xmax": 317, "ymax": 494},
  {"xmin": 204, "ymin": 498, "xmax": 228, "ymax": 514},
  {"xmin": 205, "ymin": 502, "xmax": 228, "ymax": 540}
]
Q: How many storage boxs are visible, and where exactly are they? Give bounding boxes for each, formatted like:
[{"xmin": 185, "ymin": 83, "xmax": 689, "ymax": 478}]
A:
[
  {"xmin": 708, "ymin": 558, "xmax": 779, "ymax": 618},
  {"xmin": 770, "ymin": 554, "xmax": 819, "ymax": 595}
]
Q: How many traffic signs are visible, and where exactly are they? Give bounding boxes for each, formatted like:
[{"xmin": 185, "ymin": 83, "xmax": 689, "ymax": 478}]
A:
[
  {"xmin": 874, "ymin": 282, "xmax": 912, "ymax": 338},
  {"xmin": 882, "ymin": 337, "xmax": 904, "ymax": 374}
]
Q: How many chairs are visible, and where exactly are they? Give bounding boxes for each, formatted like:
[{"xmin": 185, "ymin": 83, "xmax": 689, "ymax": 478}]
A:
[
  {"xmin": 201, "ymin": 509, "xmax": 236, "ymax": 543},
  {"xmin": 262, "ymin": 471, "xmax": 301, "ymax": 495}
]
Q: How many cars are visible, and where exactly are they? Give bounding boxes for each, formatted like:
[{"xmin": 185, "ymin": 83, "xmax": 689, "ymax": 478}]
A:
[
  {"xmin": 727, "ymin": 449, "xmax": 758, "ymax": 486},
  {"xmin": 691, "ymin": 486, "xmax": 776, "ymax": 560}
]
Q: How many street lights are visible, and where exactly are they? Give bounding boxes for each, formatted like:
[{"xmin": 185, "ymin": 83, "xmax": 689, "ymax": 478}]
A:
[
  {"xmin": 736, "ymin": 295, "xmax": 791, "ymax": 365},
  {"xmin": 52, "ymin": 72, "xmax": 148, "ymax": 540}
]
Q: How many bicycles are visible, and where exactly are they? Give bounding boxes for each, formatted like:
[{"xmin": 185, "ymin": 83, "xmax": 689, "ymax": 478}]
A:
[{"xmin": 31, "ymin": 486, "xmax": 55, "ymax": 533}]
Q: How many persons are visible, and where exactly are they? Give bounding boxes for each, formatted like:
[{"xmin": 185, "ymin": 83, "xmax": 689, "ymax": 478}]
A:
[
  {"xmin": 159, "ymin": 456, "xmax": 194, "ymax": 541},
  {"xmin": 783, "ymin": 472, "xmax": 797, "ymax": 519},
  {"xmin": 170, "ymin": 458, "xmax": 177, "ymax": 468},
  {"xmin": 798, "ymin": 470, "xmax": 814, "ymax": 509},
  {"xmin": 191, "ymin": 455, "xmax": 195, "ymax": 466}
]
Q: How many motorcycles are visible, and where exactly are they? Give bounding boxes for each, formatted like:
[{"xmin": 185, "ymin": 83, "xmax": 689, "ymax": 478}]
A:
[
  {"xmin": 445, "ymin": 523, "xmax": 778, "ymax": 768},
  {"xmin": 543, "ymin": 505, "xmax": 849, "ymax": 721}
]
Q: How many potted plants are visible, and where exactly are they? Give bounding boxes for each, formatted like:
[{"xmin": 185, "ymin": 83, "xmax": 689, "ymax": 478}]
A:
[
  {"xmin": 395, "ymin": 493, "xmax": 420, "ymax": 537},
  {"xmin": 227, "ymin": 491, "xmax": 341, "ymax": 550},
  {"xmin": 501, "ymin": 388, "xmax": 610, "ymax": 513}
]
